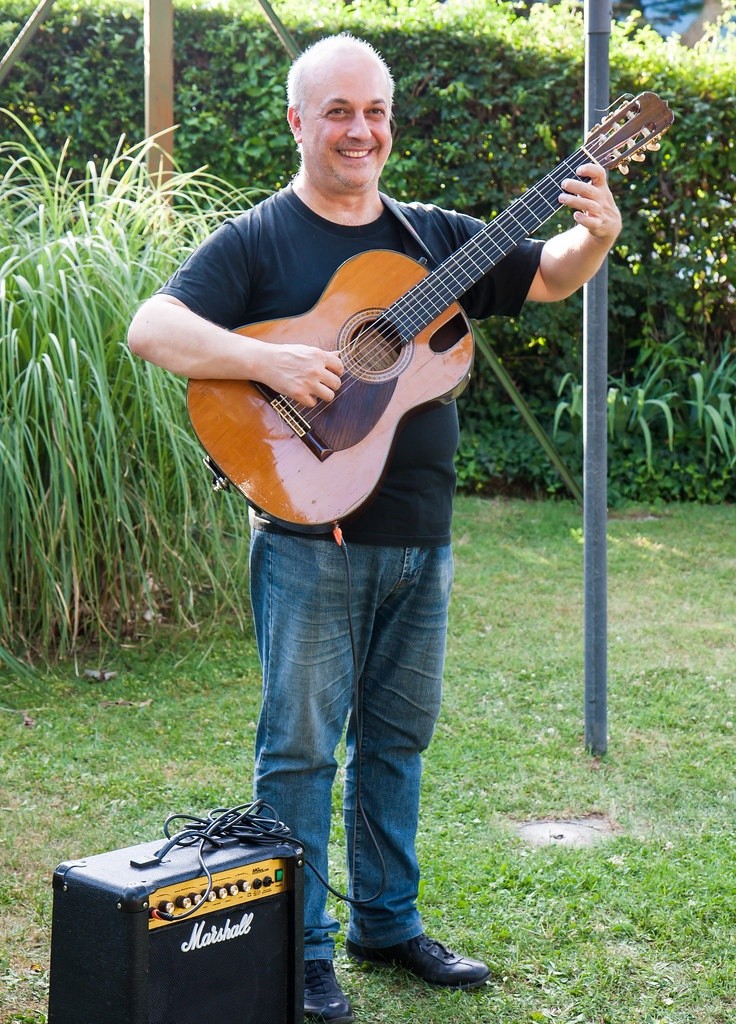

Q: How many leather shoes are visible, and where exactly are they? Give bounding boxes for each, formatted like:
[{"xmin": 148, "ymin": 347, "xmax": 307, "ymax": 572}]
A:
[
  {"xmin": 346, "ymin": 938, "xmax": 491, "ymax": 986},
  {"xmin": 304, "ymin": 960, "xmax": 354, "ymax": 1024}
]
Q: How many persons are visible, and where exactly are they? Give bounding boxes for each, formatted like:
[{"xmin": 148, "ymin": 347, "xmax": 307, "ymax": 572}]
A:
[{"xmin": 126, "ymin": 37, "xmax": 623, "ymax": 1024}]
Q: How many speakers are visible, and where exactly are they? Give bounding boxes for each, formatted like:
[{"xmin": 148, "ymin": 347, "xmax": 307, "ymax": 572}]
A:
[{"xmin": 48, "ymin": 833, "xmax": 304, "ymax": 1024}]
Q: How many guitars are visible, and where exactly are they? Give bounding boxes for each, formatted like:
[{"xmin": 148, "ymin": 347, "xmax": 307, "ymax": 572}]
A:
[{"xmin": 185, "ymin": 90, "xmax": 675, "ymax": 534}]
